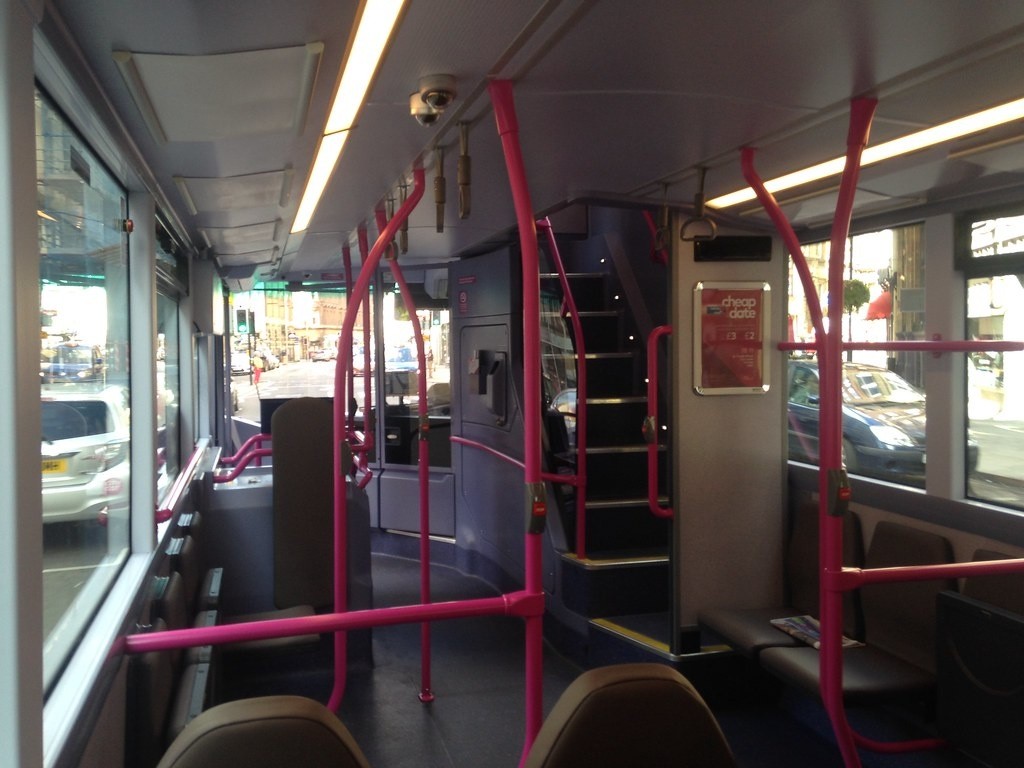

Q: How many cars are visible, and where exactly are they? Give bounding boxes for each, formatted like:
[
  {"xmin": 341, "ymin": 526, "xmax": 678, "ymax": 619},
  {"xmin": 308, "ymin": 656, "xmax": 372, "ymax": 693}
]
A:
[
  {"xmin": 49, "ymin": 346, "xmax": 107, "ymax": 387},
  {"xmin": 40, "ymin": 353, "xmax": 52, "ymax": 382},
  {"xmin": 313, "ymin": 344, "xmax": 375, "ymax": 377},
  {"xmin": 38, "ymin": 383, "xmax": 131, "ymax": 525},
  {"xmin": 230, "ymin": 348, "xmax": 280, "ymax": 372},
  {"xmin": 787, "ymin": 358, "xmax": 978, "ymax": 474}
]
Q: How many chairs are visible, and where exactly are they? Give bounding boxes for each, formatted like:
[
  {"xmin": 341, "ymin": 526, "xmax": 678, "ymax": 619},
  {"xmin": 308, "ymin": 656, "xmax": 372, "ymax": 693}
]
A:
[
  {"xmin": 125, "ymin": 510, "xmax": 373, "ymax": 768},
  {"xmin": 697, "ymin": 498, "xmax": 1024, "ymax": 768},
  {"xmin": 524, "ymin": 664, "xmax": 737, "ymax": 768}
]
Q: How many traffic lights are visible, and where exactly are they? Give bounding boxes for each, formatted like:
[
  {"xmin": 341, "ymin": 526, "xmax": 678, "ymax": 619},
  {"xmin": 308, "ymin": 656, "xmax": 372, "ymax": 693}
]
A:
[
  {"xmin": 248, "ymin": 310, "xmax": 255, "ymax": 333},
  {"xmin": 237, "ymin": 310, "xmax": 246, "ymax": 332}
]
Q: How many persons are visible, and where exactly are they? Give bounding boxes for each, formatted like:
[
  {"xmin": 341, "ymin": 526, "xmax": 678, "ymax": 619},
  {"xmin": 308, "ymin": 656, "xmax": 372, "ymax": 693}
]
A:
[
  {"xmin": 426, "ymin": 344, "xmax": 434, "ymax": 378},
  {"xmin": 253, "ymin": 351, "xmax": 264, "ymax": 383}
]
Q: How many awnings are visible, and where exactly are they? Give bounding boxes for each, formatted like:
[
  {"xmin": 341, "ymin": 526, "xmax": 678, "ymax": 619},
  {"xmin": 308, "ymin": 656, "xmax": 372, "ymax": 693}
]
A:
[{"xmin": 866, "ymin": 292, "xmax": 891, "ymax": 321}]
